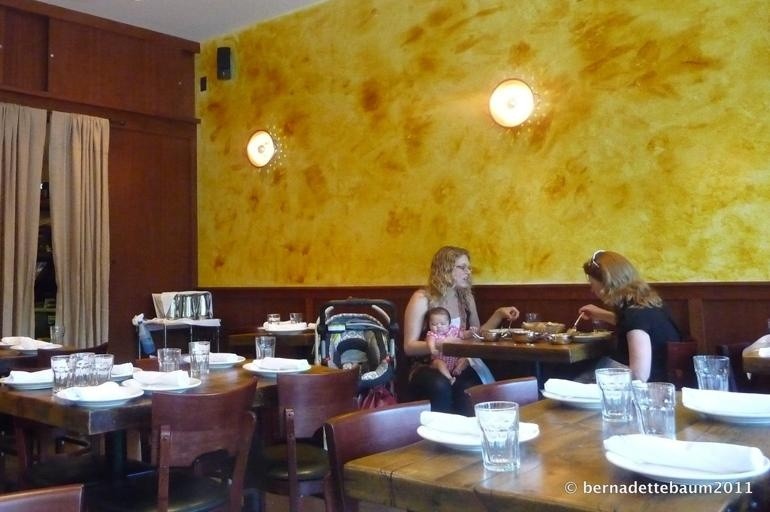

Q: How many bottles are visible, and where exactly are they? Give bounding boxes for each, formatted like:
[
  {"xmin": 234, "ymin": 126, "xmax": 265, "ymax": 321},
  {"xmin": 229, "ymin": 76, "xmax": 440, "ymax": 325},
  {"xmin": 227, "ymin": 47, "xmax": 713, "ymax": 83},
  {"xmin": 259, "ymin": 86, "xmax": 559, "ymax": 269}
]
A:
[
  {"xmin": 137, "ymin": 320, "xmax": 156, "ymax": 356},
  {"xmin": 592, "ymin": 311, "xmax": 608, "ymax": 333}
]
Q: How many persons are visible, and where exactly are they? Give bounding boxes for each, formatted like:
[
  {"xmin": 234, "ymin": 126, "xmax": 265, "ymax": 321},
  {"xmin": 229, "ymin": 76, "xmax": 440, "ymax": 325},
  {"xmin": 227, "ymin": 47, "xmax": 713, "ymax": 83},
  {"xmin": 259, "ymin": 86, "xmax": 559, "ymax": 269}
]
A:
[
  {"xmin": 577, "ymin": 249, "xmax": 684, "ymax": 383},
  {"xmin": 402, "ymin": 245, "xmax": 520, "ymax": 414},
  {"xmin": 425, "ymin": 306, "xmax": 481, "ymax": 386}
]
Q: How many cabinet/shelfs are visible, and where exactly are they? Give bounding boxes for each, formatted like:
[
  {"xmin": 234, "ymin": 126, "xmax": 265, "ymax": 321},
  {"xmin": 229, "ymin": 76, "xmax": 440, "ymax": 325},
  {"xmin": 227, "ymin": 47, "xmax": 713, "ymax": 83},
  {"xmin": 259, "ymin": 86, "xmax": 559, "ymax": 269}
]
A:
[{"xmin": 1, "ymin": 6, "xmax": 196, "ymax": 122}]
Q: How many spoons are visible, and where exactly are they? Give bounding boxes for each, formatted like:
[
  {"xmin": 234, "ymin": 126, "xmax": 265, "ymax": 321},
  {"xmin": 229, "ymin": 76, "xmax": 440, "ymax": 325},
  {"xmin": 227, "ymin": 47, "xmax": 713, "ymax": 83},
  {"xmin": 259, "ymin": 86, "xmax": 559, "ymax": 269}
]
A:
[{"xmin": 571, "ymin": 312, "xmax": 584, "ymax": 331}]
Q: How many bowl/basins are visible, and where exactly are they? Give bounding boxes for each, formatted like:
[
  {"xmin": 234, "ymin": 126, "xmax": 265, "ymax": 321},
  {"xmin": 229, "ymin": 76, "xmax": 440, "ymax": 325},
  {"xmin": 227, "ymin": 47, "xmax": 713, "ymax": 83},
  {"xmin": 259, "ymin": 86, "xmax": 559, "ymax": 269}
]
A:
[
  {"xmin": 522, "ymin": 321, "xmax": 564, "ymax": 334},
  {"xmin": 475, "ymin": 328, "xmax": 571, "ymax": 345}
]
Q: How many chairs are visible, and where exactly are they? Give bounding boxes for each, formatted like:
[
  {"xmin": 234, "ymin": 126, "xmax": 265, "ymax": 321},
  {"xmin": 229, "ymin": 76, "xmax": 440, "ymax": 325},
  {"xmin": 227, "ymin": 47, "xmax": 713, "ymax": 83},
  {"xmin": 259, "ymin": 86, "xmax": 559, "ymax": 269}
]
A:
[{"xmin": 0, "ymin": 328, "xmax": 766, "ymax": 511}]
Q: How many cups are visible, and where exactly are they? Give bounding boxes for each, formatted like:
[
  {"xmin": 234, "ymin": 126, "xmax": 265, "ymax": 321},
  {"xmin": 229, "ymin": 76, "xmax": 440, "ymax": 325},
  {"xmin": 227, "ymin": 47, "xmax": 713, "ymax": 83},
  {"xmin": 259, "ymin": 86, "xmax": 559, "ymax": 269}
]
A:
[
  {"xmin": 267, "ymin": 313, "xmax": 281, "ymax": 326},
  {"xmin": 693, "ymin": 353, "xmax": 730, "ymax": 393},
  {"xmin": 49, "ymin": 325, "xmax": 65, "ymax": 345},
  {"xmin": 594, "ymin": 366, "xmax": 633, "ymax": 423},
  {"xmin": 188, "ymin": 341, "xmax": 211, "ymax": 377},
  {"xmin": 473, "ymin": 400, "xmax": 521, "ymax": 474},
  {"xmin": 631, "ymin": 380, "xmax": 678, "ymax": 440},
  {"xmin": 50, "ymin": 351, "xmax": 114, "ymax": 392},
  {"xmin": 255, "ymin": 335, "xmax": 276, "ymax": 358},
  {"xmin": 289, "ymin": 312, "xmax": 304, "ymax": 323},
  {"xmin": 525, "ymin": 313, "xmax": 541, "ymax": 322},
  {"xmin": 157, "ymin": 347, "xmax": 183, "ymax": 372}
]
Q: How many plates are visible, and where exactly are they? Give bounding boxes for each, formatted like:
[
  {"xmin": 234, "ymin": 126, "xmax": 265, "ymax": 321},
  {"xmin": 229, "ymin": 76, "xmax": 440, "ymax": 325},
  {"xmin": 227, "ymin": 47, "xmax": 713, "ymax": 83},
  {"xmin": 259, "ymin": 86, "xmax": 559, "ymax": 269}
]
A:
[
  {"xmin": 54, "ymin": 387, "xmax": 144, "ymax": 407},
  {"xmin": 417, "ymin": 422, "xmax": 540, "ymax": 453},
  {"xmin": 0, "ymin": 339, "xmax": 48, "ymax": 347},
  {"xmin": 1, "ymin": 376, "xmax": 52, "ymax": 389},
  {"xmin": 539, "ymin": 390, "xmax": 602, "ymax": 414},
  {"xmin": 684, "ymin": 402, "xmax": 770, "ymax": 426},
  {"xmin": 111, "ymin": 365, "xmax": 141, "ymax": 382},
  {"xmin": 573, "ymin": 332, "xmax": 613, "ymax": 343},
  {"xmin": 9, "ymin": 344, "xmax": 63, "ymax": 354},
  {"xmin": 184, "ymin": 355, "xmax": 246, "ymax": 368},
  {"xmin": 243, "ymin": 362, "xmax": 313, "ymax": 379},
  {"xmin": 122, "ymin": 378, "xmax": 202, "ymax": 394},
  {"xmin": 604, "ymin": 449, "xmax": 770, "ymax": 486}
]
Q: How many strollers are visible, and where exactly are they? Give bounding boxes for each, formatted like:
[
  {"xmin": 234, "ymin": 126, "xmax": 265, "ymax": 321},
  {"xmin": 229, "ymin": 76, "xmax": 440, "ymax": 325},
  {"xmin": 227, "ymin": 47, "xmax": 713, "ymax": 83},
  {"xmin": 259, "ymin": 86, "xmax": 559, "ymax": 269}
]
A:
[{"xmin": 310, "ymin": 296, "xmax": 399, "ymax": 450}]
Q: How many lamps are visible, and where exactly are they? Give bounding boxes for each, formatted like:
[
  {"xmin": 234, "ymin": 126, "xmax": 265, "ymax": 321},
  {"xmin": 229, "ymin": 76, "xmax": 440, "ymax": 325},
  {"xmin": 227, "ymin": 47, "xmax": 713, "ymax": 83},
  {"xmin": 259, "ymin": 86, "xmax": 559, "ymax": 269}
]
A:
[
  {"xmin": 488, "ymin": 80, "xmax": 534, "ymax": 129},
  {"xmin": 246, "ymin": 130, "xmax": 273, "ymax": 167}
]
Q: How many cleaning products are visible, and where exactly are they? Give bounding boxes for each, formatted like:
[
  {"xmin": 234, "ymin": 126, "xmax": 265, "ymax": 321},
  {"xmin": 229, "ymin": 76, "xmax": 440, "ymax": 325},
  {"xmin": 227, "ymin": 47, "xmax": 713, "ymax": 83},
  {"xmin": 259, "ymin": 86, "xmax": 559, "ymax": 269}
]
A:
[{"xmin": 133, "ymin": 312, "xmax": 156, "ymax": 354}]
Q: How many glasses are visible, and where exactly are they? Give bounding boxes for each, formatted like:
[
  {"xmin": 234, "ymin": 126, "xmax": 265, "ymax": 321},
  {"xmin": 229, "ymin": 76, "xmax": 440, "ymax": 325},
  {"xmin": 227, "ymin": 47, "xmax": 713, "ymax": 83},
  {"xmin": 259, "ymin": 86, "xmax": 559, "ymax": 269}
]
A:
[{"xmin": 592, "ymin": 250, "xmax": 605, "ymax": 267}]
